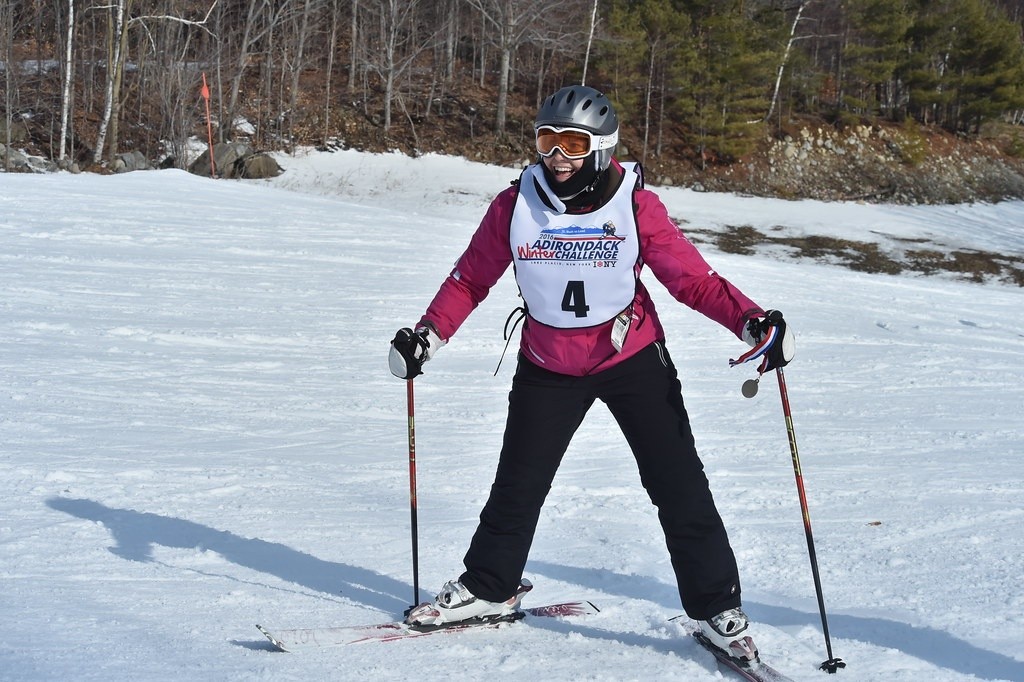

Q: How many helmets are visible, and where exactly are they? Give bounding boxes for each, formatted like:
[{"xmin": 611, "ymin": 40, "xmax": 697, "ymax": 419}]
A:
[{"xmin": 535, "ymin": 85, "xmax": 619, "ymax": 172}]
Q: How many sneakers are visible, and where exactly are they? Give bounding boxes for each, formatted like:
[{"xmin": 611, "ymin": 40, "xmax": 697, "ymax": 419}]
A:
[
  {"xmin": 697, "ymin": 607, "xmax": 758, "ymax": 661},
  {"xmin": 408, "ymin": 578, "xmax": 506, "ymax": 626}
]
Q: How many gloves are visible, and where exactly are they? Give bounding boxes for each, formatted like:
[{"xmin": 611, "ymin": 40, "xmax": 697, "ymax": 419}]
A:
[
  {"xmin": 389, "ymin": 328, "xmax": 430, "ymax": 379},
  {"xmin": 747, "ymin": 309, "xmax": 795, "ymax": 367}
]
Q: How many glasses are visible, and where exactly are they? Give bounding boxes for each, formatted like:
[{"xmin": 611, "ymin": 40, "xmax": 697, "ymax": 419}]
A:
[{"xmin": 535, "ymin": 126, "xmax": 619, "ymax": 159}]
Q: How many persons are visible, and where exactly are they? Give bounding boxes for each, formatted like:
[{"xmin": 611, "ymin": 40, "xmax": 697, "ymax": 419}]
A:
[{"xmin": 388, "ymin": 84, "xmax": 797, "ymax": 664}]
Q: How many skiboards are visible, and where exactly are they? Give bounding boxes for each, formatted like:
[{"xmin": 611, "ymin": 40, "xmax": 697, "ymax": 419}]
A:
[{"xmin": 252, "ymin": 596, "xmax": 797, "ymax": 681}]
